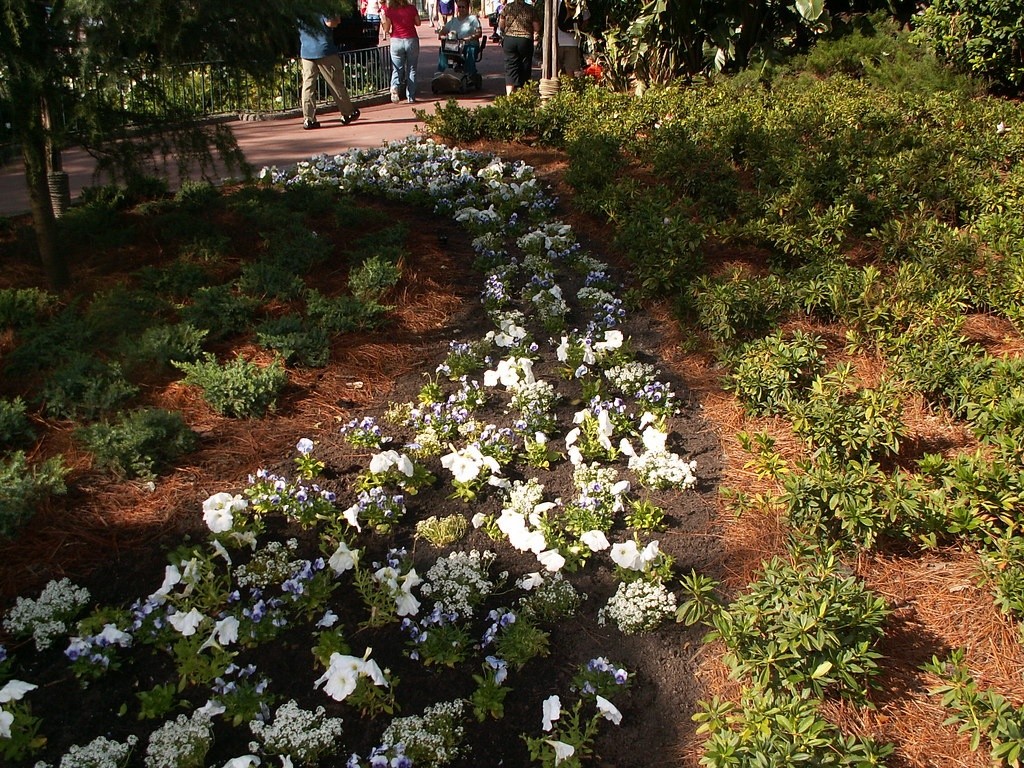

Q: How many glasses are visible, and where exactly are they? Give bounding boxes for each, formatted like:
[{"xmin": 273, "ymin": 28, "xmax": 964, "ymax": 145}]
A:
[{"xmin": 458, "ymin": 6, "xmax": 466, "ymax": 9}]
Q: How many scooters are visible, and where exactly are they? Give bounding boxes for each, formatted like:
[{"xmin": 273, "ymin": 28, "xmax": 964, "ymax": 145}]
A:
[{"xmin": 430, "ymin": 28, "xmax": 487, "ymax": 93}]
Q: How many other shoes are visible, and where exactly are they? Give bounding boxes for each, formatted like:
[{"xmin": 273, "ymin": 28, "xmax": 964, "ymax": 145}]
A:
[
  {"xmin": 303, "ymin": 120, "xmax": 320, "ymax": 129},
  {"xmin": 390, "ymin": 84, "xmax": 399, "ymax": 104},
  {"xmin": 407, "ymin": 97, "xmax": 414, "ymax": 103},
  {"xmin": 342, "ymin": 109, "xmax": 360, "ymax": 124}
]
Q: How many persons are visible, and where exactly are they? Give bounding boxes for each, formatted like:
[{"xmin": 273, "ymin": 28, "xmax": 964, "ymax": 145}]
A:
[
  {"xmin": 385, "ymin": 0, "xmax": 421, "ymax": 104},
  {"xmin": 436, "ymin": 0, "xmax": 483, "ymax": 89},
  {"xmin": 492, "ymin": 0, "xmax": 508, "ymax": 37},
  {"xmin": 358, "ymin": 0, "xmax": 391, "ymax": 40},
  {"xmin": 424, "ymin": 0, "xmax": 458, "ymax": 29},
  {"xmin": 296, "ymin": 14, "xmax": 360, "ymax": 130},
  {"xmin": 557, "ymin": 0, "xmax": 590, "ymax": 75},
  {"xmin": 499, "ymin": 0, "xmax": 541, "ymax": 98}
]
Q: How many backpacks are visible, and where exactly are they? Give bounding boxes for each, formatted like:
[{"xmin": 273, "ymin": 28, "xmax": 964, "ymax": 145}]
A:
[{"xmin": 557, "ymin": 0, "xmax": 583, "ymax": 33}]
